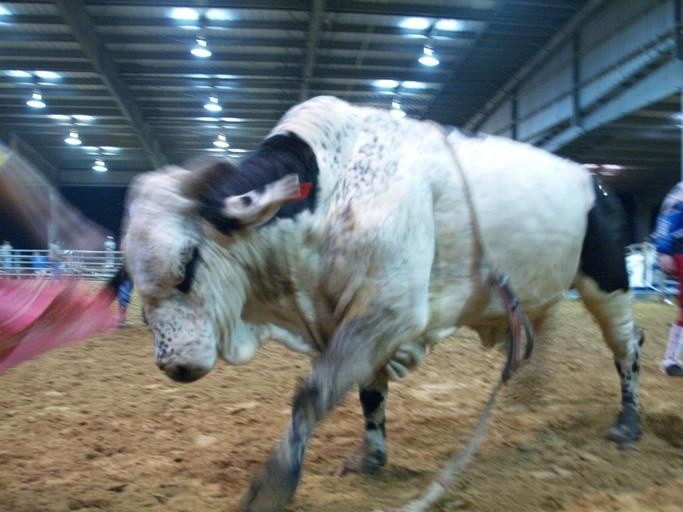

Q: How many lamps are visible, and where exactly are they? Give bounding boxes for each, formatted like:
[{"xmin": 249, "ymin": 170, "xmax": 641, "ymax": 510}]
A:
[
  {"xmin": 204, "ymin": 92, "xmax": 223, "ymax": 113},
  {"xmin": 211, "ymin": 132, "xmax": 231, "ymax": 149},
  {"xmin": 416, "ymin": 43, "xmax": 442, "ymax": 68},
  {"xmin": 60, "ymin": 128, "xmax": 84, "ymax": 146},
  {"xmin": 92, "ymin": 159, "xmax": 109, "ymax": 174},
  {"xmin": 187, "ymin": 33, "xmax": 214, "ymax": 59},
  {"xmin": 25, "ymin": 90, "xmax": 48, "ymax": 111}
]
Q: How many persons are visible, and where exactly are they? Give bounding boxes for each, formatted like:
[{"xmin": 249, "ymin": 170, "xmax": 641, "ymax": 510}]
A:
[
  {"xmin": 654, "ymin": 179, "xmax": 682, "ymax": 377},
  {"xmin": 49, "ymin": 239, "xmax": 66, "ymax": 285},
  {"xmin": 115, "ymin": 282, "xmax": 133, "ymax": 328},
  {"xmin": 1, "ymin": 240, "xmax": 13, "ymax": 273},
  {"xmin": 31, "ymin": 252, "xmax": 47, "ymax": 280},
  {"xmin": 103, "ymin": 235, "xmax": 117, "ymax": 267}
]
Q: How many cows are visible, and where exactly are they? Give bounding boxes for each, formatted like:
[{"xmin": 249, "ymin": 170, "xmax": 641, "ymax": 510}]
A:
[{"xmin": 107, "ymin": 95, "xmax": 645, "ymax": 512}]
